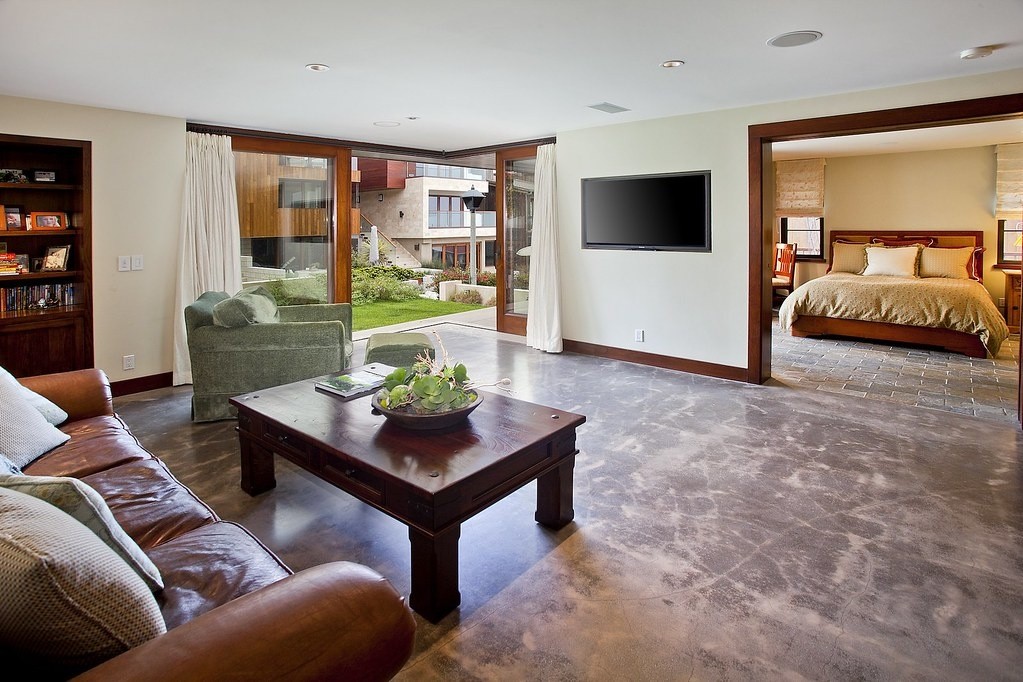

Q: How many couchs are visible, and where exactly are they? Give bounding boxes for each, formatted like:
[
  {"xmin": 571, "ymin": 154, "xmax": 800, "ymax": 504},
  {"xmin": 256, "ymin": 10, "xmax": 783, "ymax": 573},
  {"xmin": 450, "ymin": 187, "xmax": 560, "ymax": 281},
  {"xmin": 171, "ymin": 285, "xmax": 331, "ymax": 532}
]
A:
[
  {"xmin": 16, "ymin": 367, "xmax": 420, "ymax": 682},
  {"xmin": 186, "ymin": 289, "xmax": 353, "ymax": 421}
]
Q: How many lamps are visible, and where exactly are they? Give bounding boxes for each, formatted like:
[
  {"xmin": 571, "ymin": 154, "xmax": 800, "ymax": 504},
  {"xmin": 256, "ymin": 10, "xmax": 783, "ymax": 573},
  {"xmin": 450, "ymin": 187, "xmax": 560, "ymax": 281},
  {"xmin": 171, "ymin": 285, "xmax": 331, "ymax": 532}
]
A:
[
  {"xmin": 379, "ymin": 193, "xmax": 383, "ymax": 200},
  {"xmin": 356, "ymin": 195, "xmax": 360, "ymax": 202}
]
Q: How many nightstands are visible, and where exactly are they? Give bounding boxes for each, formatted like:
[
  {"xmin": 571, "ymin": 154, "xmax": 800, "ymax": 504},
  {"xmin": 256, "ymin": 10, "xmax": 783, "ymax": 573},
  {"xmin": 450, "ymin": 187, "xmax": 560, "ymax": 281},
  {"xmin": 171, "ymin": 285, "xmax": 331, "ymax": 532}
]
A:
[{"xmin": 1001, "ymin": 268, "xmax": 1022, "ymax": 333}]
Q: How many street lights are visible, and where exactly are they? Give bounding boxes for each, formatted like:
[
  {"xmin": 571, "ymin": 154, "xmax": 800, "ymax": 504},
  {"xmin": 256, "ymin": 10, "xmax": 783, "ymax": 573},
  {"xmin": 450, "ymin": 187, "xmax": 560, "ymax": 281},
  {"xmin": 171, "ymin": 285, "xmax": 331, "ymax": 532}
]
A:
[{"xmin": 461, "ymin": 183, "xmax": 487, "ymax": 285}]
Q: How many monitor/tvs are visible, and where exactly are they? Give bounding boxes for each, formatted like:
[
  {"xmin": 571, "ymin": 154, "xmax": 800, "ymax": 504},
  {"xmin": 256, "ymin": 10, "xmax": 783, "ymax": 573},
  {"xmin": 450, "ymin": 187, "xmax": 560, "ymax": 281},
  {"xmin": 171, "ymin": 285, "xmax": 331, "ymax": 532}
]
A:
[{"xmin": 583, "ymin": 170, "xmax": 712, "ymax": 252}]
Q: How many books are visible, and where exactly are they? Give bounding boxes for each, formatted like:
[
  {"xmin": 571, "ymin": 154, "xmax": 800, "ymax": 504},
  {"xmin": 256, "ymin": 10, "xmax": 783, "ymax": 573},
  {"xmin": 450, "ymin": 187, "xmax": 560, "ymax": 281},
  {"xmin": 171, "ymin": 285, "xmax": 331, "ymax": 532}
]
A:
[
  {"xmin": 0, "ymin": 252, "xmax": 29, "ymax": 276},
  {"xmin": 315, "ymin": 370, "xmax": 386, "ymax": 398},
  {"xmin": 0, "ymin": 283, "xmax": 73, "ymax": 312}
]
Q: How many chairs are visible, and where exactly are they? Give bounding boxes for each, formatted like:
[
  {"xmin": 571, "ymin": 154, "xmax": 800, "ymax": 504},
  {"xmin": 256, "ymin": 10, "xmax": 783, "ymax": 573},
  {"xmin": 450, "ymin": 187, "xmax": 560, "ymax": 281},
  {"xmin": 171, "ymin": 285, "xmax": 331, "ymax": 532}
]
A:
[{"xmin": 771, "ymin": 240, "xmax": 797, "ymax": 314}]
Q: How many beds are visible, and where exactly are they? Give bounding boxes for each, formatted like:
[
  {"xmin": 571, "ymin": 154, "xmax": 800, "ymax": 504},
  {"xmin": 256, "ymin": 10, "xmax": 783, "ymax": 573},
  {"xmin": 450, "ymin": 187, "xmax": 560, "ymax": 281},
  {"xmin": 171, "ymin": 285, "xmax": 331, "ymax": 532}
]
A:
[{"xmin": 778, "ymin": 230, "xmax": 1009, "ymax": 360}]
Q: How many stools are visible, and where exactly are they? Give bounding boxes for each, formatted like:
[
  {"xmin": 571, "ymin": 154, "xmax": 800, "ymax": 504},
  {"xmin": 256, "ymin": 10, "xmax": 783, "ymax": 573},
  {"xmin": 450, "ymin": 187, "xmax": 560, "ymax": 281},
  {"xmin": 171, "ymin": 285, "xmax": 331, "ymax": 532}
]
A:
[{"xmin": 363, "ymin": 332, "xmax": 434, "ymax": 367}]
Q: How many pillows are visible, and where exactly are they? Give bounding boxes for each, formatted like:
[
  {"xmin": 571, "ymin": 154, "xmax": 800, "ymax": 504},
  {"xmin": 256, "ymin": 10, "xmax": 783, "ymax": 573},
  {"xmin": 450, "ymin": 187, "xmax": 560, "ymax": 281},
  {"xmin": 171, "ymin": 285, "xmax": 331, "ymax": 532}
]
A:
[
  {"xmin": 0, "ymin": 365, "xmax": 68, "ymax": 426},
  {"xmin": 210, "ymin": 284, "xmax": 280, "ymax": 326},
  {"xmin": 917, "ymin": 245, "xmax": 988, "ymax": 283},
  {"xmin": 826, "ymin": 240, "xmax": 885, "ymax": 275},
  {"xmin": 0, "ymin": 472, "xmax": 165, "ymax": 588},
  {"xmin": 856, "ymin": 244, "xmax": 925, "ymax": 279},
  {"xmin": 0, "ymin": 379, "xmax": 71, "ymax": 471},
  {"xmin": 0, "ymin": 486, "xmax": 164, "ymax": 670}
]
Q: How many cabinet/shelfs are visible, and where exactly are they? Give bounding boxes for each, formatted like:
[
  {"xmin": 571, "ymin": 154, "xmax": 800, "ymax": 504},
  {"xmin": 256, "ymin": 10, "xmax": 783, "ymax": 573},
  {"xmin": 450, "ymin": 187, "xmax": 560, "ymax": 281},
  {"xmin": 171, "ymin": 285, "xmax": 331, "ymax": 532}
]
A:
[{"xmin": 0, "ymin": 131, "xmax": 95, "ymax": 379}]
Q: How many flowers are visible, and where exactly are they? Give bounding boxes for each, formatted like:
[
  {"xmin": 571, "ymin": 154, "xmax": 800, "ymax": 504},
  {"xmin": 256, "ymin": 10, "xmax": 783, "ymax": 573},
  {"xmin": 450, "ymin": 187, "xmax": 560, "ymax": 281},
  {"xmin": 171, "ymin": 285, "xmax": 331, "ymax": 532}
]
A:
[{"xmin": 375, "ymin": 329, "xmax": 514, "ymax": 409}]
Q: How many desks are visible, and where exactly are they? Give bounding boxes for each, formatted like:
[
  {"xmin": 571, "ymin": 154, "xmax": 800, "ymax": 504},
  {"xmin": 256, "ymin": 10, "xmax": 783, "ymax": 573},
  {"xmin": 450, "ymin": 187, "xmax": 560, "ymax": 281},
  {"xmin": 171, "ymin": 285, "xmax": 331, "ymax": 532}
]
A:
[{"xmin": 229, "ymin": 361, "xmax": 588, "ymax": 626}]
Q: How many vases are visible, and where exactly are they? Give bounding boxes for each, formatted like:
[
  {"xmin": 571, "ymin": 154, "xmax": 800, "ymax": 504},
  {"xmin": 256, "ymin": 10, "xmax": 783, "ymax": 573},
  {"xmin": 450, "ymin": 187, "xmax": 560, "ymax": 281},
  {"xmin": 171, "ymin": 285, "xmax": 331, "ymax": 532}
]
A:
[{"xmin": 371, "ymin": 379, "xmax": 484, "ymax": 430}]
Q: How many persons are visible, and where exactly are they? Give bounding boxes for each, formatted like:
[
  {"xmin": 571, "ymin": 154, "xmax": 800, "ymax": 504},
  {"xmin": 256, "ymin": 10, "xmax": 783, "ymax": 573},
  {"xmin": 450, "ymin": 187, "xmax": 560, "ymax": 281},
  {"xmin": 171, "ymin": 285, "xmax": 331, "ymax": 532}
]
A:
[
  {"xmin": 35, "ymin": 260, "xmax": 41, "ymax": 270},
  {"xmin": 41, "ymin": 216, "xmax": 56, "ymax": 227},
  {"xmin": 7, "ymin": 215, "xmax": 17, "ymax": 226},
  {"xmin": 46, "ymin": 251, "xmax": 61, "ymax": 268},
  {"xmin": 13, "ymin": 174, "xmax": 26, "ymax": 183}
]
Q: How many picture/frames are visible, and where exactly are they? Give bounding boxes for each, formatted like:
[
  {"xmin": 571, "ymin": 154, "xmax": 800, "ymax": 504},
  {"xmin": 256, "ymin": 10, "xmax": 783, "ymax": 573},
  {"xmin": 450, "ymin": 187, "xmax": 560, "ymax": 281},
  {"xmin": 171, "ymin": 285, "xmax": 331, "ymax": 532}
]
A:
[
  {"xmin": 41, "ymin": 244, "xmax": 70, "ymax": 271},
  {"xmin": 30, "ymin": 211, "xmax": 66, "ymax": 230}
]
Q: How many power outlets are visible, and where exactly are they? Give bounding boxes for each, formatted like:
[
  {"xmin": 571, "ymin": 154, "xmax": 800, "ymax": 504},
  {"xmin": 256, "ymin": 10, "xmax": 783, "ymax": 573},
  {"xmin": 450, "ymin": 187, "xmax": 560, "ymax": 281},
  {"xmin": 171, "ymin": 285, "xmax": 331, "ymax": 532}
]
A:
[
  {"xmin": 635, "ymin": 329, "xmax": 644, "ymax": 342},
  {"xmin": 122, "ymin": 354, "xmax": 135, "ymax": 369}
]
